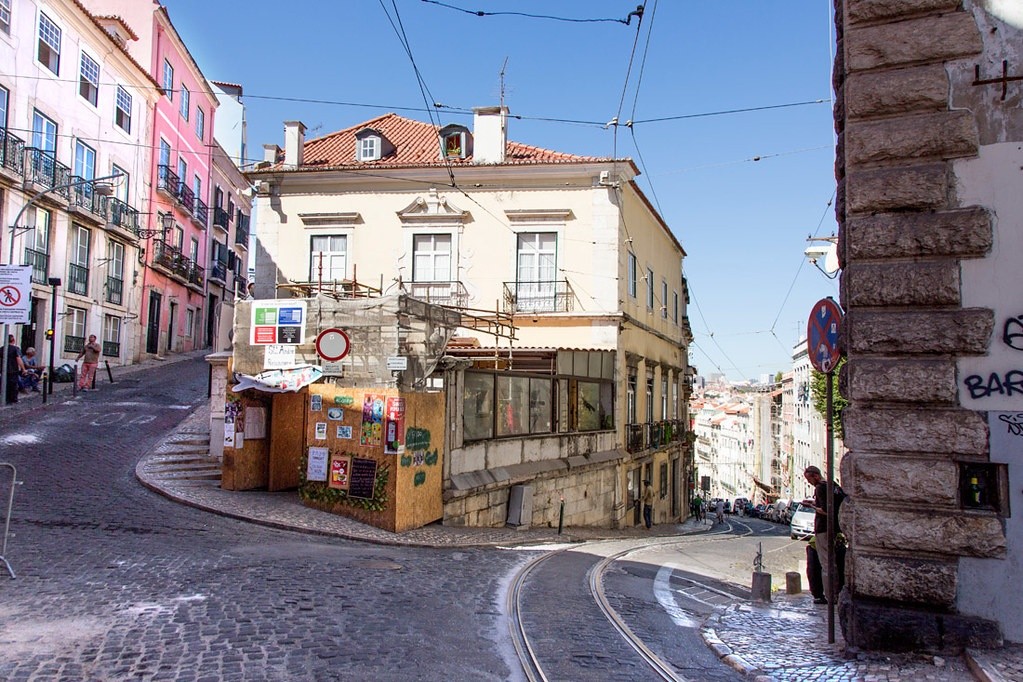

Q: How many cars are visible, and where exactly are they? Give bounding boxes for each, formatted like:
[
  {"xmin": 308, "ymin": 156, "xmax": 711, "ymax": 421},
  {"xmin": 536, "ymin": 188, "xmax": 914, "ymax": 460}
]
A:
[
  {"xmin": 701, "ymin": 498, "xmax": 725, "ymax": 512},
  {"xmin": 747, "ymin": 504, "xmax": 774, "ymax": 520}
]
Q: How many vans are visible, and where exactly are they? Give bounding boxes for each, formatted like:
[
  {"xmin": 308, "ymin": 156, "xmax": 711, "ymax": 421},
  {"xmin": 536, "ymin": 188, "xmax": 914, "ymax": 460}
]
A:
[{"xmin": 733, "ymin": 498, "xmax": 749, "ymax": 515}]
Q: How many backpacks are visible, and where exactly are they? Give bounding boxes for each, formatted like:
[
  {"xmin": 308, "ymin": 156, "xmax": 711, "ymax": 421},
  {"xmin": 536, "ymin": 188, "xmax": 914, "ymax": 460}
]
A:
[{"xmin": 819, "ymin": 482, "xmax": 845, "ymax": 511}]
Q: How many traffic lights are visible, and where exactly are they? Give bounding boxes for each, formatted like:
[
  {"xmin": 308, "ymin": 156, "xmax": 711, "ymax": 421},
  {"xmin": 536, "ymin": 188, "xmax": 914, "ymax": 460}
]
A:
[{"xmin": 47, "ymin": 330, "xmax": 54, "ymax": 341}]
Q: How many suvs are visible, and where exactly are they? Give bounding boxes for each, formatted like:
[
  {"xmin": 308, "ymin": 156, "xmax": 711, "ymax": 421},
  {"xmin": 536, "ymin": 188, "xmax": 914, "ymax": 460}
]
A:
[
  {"xmin": 782, "ymin": 500, "xmax": 803, "ymax": 525},
  {"xmin": 791, "ymin": 495, "xmax": 817, "ymax": 540},
  {"xmin": 772, "ymin": 498, "xmax": 790, "ymax": 523}
]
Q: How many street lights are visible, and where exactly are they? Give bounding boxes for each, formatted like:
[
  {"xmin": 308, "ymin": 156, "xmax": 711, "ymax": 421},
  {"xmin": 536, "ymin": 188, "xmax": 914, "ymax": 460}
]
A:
[
  {"xmin": 46, "ymin": 277, "xmax": 61, "ymax": 396},
  {"xmin": 1, "ymin": 173, "xmax": 125, "ymax": 408}
]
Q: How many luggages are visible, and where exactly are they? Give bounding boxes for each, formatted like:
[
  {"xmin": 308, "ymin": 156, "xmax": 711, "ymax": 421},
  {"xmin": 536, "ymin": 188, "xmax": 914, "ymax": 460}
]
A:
[{"xmin": 806, "ymin": 542, "xmax": 846, "ymax": 599}]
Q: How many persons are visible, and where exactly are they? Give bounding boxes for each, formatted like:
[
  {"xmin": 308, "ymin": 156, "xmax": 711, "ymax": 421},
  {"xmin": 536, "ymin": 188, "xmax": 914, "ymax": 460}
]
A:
[
  {"xmin": 638, "ymin": 480, "xmax": 655, "ymax": 529},
  {"xmin": 0, "ymin": 334, "xmax": 25, "ymax": 404},
  {"xmin": 693, "ymin": 494, "xmax": 703, "ymax": 521},
  {"xmin": 802, "ymin": 465, "xmax": 845, "ymax": 605},
  {"xmin": 739, "ymin": 500, "xmax": 753, "ymax": 518},
  {"xmin": 18, "ymin": 347, "xmax": 46, "ymax": 394},
  {"xmin": 75, "ymin": 334, "xmax": 101, "ymax": 392},
  {"xmin": 715, "ymin": 499, "xmax": 731, "ymax": 524}
]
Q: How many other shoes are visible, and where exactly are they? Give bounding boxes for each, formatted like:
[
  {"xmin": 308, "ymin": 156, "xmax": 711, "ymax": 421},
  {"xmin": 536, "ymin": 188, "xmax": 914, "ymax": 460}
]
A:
[{"xmin": 814, "ymin": 597, "xmax": 828, "ymax": 604}]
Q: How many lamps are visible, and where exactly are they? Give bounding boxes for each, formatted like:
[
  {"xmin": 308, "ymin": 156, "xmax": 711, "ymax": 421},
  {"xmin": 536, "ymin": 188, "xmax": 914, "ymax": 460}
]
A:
[{"xmin": 138, "ymin": 211, "xmax": 176, "ymax": 238}]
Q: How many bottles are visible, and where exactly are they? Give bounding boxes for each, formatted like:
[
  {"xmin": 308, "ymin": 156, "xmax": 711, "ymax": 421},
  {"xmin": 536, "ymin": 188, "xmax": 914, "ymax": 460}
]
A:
[{"xmin": 969, "ymin": 473, "xmax": 981, "ymax": 506}]
